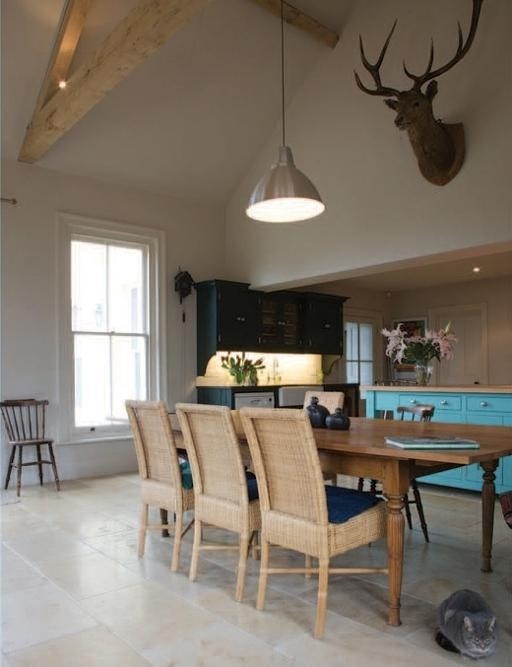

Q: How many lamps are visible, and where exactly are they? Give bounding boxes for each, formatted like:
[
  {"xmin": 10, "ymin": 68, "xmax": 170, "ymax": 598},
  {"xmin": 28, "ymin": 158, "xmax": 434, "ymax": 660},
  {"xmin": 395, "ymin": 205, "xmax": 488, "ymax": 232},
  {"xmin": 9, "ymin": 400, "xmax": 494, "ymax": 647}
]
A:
[{"xmin": 246, "ymin": 0, "xmax": 325, "ymax": 224}]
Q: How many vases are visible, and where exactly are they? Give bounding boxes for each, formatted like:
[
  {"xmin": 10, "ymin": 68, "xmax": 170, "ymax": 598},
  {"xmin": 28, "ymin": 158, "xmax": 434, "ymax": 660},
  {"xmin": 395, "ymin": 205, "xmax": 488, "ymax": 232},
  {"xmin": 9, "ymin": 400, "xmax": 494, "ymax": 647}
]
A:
[{"xmin": 415, "ymin": 365, "xmax": 433, "ymax": 385}]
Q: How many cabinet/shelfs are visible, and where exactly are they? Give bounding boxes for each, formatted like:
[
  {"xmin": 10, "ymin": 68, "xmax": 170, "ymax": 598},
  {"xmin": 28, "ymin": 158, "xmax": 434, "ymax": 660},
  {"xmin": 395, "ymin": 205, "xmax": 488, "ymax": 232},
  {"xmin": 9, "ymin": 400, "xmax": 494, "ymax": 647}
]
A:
[
  {"xmin": 398, "ymin": 393, "xmax": 512, "ymax": 495},
  {"xmin": 192, "ymin": 278, "xmax": 351, "ymax": 356}
]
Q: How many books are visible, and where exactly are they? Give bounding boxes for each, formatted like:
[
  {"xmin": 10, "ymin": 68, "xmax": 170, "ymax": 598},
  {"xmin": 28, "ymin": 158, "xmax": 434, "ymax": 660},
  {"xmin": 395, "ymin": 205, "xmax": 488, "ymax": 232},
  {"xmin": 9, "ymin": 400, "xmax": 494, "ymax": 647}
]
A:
[{"xmin": 384, "ymin": 430, "xmax": 480, "ymax": 452}]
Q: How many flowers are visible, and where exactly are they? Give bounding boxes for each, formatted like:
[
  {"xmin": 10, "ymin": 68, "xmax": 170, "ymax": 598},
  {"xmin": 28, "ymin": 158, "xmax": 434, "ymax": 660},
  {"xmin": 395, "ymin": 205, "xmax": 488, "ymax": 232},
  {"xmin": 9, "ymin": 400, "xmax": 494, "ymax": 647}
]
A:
[
  {"xmin": 379, "ymin": 320, "xmax": 459, "ymax": 366},
  {"xmin": 219, "ymin": 352, "xmax": 265, "ymax": 384}
]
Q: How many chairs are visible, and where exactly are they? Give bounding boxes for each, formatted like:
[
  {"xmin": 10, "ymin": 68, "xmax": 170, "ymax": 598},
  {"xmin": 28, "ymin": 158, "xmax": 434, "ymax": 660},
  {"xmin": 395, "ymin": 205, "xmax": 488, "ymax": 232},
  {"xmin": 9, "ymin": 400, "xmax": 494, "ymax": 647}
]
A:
[
  {"xmin": 126, "ymin": 398, "xmax": 204, "ymax": 571},
  {"xmin": 174, "ymin": 402, "xmax": 261, "ymax": 602},
  {"xmin": 239, "ymin": 407, "xmax": 387, "ymax": 640},
  {"xmin": 1, "ymin": 397, "xmax": 62, "ymax": 498}
]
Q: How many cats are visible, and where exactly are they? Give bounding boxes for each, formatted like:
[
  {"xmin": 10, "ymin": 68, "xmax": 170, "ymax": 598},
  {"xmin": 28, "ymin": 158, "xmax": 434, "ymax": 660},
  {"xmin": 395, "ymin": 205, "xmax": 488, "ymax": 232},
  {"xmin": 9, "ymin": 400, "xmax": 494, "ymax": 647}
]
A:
[{"xmin": 435, "ymin": 590, "xmax": 496, "ymax": 659}]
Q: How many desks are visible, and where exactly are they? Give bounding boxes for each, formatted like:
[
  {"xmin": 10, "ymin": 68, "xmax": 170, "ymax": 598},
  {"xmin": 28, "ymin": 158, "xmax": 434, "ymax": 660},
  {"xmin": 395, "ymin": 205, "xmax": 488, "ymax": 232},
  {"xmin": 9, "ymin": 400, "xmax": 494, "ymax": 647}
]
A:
[{"xmin": 106, "ymin": 413, "xmax": 511, "ymax": 626}]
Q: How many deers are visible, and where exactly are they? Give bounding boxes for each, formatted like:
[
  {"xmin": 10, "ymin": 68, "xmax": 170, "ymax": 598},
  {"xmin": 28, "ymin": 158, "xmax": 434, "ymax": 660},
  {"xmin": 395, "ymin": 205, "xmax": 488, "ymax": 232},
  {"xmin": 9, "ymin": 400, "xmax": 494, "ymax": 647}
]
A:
[{"xmin": 350, "ymin": 1, "xmax": 483, "ymax": 185}]
